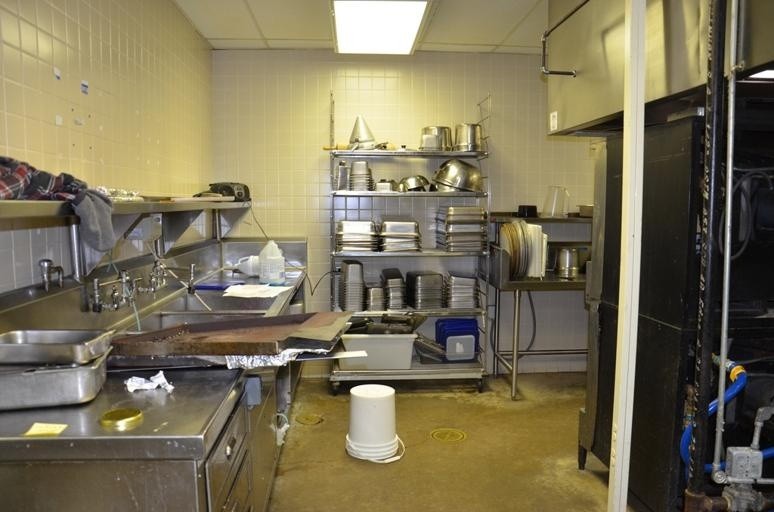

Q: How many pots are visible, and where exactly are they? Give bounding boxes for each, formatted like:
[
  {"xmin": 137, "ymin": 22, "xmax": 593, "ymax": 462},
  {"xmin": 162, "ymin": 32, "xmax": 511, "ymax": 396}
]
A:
[
  {"xmin": 395, "ymin": 123, "xmax": 483, "ymax": 192},
  {"xmin": 554, "ymin": 246, "xmax": 590, "ymax": 280}
]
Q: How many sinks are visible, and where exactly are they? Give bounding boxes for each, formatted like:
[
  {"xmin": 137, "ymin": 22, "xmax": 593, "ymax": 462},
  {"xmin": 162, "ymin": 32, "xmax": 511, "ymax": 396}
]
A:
[
  {"xmin": 118, "ymin": 309, "xmax": 262, "ymax": 337},
  {"xmin": 152, "ymin": 281, "xmax": 280, "ymax": 313}
]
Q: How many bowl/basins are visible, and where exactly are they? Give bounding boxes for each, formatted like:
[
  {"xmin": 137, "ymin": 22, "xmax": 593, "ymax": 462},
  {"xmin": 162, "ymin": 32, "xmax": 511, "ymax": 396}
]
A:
[{"xmin": 576, "ymin": 204, "xmax": 594, "ymax": 217}]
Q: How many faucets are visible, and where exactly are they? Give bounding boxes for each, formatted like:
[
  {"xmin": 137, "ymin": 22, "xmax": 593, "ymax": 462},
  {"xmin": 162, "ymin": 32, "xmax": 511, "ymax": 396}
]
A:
[
  {"xmin": 106, "ymin": 267, "xmax": 139, "ymax": 310},
  {"xmin": 150, "ymin": 261, "xmax": 199, "ymax": 295}
]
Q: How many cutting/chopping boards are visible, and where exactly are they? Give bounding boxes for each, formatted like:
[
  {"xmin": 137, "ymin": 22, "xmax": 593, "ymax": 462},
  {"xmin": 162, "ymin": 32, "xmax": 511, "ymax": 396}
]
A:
[{"xmin": 527, "ymin": 223, "xmax": 548, "ymax": 279}]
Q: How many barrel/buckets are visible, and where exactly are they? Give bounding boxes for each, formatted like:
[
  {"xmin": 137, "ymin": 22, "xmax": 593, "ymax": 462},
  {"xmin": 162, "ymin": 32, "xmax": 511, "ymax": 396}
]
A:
[
  {"xmin": 454, "ymin": 124, "xmax": 483, "ymax": 153},
  {"xmin": 233, "ymin": 255, "xmax": 259, "ymax": 276},
  {"xmin": 345, "ymin": 383, "xmax": 405, "ymax": 464},
  {"xmin": 259, "ymin": 238, "xmax": 286, "ymax": 285}
]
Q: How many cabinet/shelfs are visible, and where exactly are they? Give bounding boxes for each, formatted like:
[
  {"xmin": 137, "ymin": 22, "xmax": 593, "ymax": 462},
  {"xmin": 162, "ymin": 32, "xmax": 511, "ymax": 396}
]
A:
[
  {"xmin": 333, "ymin": 152, "xmax": 487, "ymax": 378},
  {"xmin": 0, "ymin": 387, "xmax": 255, "ymax": 511}
]
